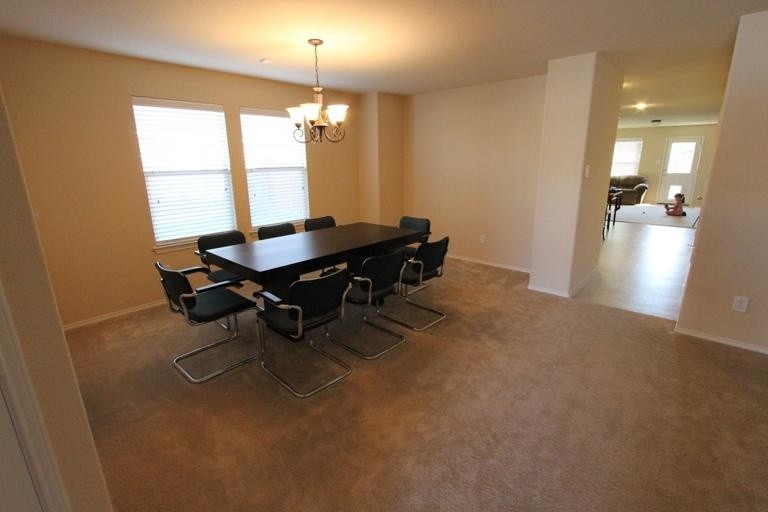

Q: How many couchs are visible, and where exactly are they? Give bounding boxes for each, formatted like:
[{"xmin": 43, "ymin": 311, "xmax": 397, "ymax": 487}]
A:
[{"xmin": 608, "ymin": 175, "xmax": 649, "ymax": 205}]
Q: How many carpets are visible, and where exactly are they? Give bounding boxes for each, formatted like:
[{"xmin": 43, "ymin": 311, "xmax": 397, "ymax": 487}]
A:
[{"xmin": 606, "ymin": 202, "xmax": 701, "ymax": 228}]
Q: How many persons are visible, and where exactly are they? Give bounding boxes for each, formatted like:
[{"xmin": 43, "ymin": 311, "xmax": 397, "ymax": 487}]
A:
[{"xmin": 665, "ymin": 193, "xmax": 684, "ymax": 216}]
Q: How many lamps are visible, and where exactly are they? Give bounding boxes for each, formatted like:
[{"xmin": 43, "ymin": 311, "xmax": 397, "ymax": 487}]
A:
[{"xmin": 285, "ymin": 39, "xmax": 350, "ymax": 144}]
